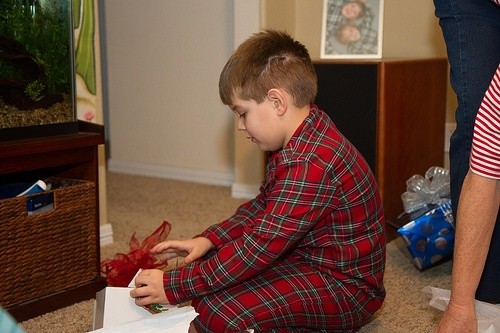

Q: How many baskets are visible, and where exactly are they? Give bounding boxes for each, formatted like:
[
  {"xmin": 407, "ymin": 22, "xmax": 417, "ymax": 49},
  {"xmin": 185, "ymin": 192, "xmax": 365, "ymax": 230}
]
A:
[{"xmin": 0, "ymin": 176, "xmax": 99, "ymax": 308}]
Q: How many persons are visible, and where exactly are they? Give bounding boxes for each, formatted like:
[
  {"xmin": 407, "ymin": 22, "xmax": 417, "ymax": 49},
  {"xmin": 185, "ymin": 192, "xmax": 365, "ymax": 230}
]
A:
[
  {"xmin": 435, "ymin": 59, "xmax": 500, "ymax": 333},
  {"xmin": 334, "ymin": 5, "xmax": 379, "ymax": 55},
  {"xmin": 434, "ymin": 1, "xmax": 500, "ymax": 304},
  {"xmin": 324, "ymin": 1, "xmax": 373, "ymax": 56},
  {"xmin": 130, "ymin": 30, "xmax": 390, "ymax": 333}
]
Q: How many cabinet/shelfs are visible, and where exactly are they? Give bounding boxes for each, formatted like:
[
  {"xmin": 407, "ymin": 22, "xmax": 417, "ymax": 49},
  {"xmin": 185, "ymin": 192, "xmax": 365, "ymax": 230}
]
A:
[{"xmin": 313, "ymin": 56, "xmax": 448, "ymax": 247}]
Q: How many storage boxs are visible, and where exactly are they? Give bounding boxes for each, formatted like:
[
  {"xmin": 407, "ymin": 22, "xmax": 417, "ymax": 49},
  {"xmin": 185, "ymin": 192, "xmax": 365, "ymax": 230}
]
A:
[{"xmin": 0, "ymin": 179, "xmax": 100, "ymax": 311}]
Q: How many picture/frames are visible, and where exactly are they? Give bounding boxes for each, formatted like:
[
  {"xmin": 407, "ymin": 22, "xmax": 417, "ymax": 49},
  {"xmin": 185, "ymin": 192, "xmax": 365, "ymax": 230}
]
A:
[{"xmin": 319, "ymin": 0, "xmax": 386, "ymax": 61}]
